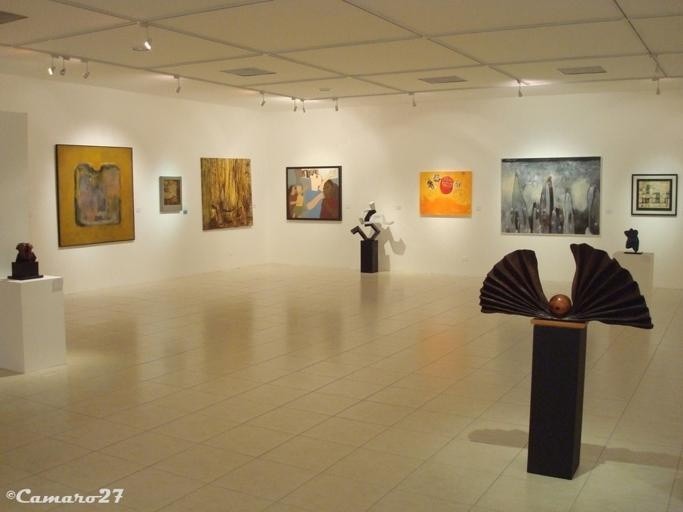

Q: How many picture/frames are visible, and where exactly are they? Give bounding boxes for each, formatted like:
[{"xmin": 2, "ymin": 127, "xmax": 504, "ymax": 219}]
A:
[
  {"xmin": 631, "ymin": 174, "xmax": 678, "ymax": 216},
  {"xmin": 286, "ymin": 166, "xmax": 342, "ymax": 221},
  {"xmin": 55, "ymin": 144, "xmax": 135, "ymax": 247},
  {"xmin": 159, "ymin": 175, "xmax": 182, "ymax": 213}
]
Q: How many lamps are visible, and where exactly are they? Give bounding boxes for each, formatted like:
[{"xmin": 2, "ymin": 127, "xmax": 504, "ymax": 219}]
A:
[
  {"xmin": 333, "ymin": 97, "xmax": 339, "ymax": 111},
  {"xmin": 173, "ymin": 74, "xmax": 182, "ymax": 94},
  {"xmin": 259, "ymin": 90, "xmax": 266, "ymax": 107},
  {"xmin": 132, "ymin": 20, "xmax": 153, "ymax": 52},
  {"xmin": 300, "ymin": 98, "xmax": 306, "ymax": 113},
  {"xmin": 652, "ymin": 77, "xmax": 661, "ymax": 95},
  {"xmin": 408, "ymin": 92, "xmax": 416, "ymax": 107},
  {"xmin": 291, "ymin": 96, "xmax": 298, "ymax": 112},
  {"xmin": 47, "ymin": 53, "xmax": 90, "ymax": 79},
  {"xmin": 517, "ymin": 80, "xmax": 523, "ymax": 97}
]
